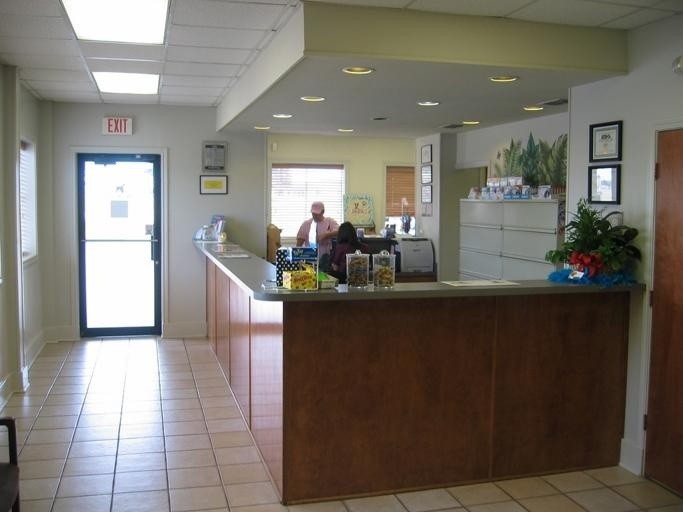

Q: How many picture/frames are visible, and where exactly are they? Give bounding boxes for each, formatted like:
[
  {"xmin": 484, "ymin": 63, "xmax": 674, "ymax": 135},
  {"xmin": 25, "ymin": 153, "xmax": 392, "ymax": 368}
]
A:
[
  {"xmin": 418, "ymin": 143, "xmax": 433, "ymax": 203},
  {"xmin": 198, "ymin": 174, "xmax": 228, "ymax": 195},
  {"xmin": 587, "ymin": 121, "xmax": 622, "ymax": 204}
]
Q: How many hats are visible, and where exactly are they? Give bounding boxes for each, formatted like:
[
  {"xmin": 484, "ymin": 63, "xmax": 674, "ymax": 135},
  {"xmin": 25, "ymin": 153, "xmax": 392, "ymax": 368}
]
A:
[{"xmin": 311, "ymin": 202, "xmax": 324, "ymax": 215}]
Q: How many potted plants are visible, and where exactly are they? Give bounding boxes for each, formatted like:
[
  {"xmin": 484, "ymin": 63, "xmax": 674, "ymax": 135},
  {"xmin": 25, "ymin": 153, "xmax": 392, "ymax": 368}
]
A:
[{"xmin": 502, "ymin": 135, "xmax": 568, "ymax": 199}]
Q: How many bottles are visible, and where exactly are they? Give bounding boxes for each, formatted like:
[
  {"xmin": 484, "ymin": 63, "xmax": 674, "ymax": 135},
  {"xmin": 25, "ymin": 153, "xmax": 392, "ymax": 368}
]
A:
[{"xmin": 346, "ymin": 249, "xmax": 397, "ymax": 292}]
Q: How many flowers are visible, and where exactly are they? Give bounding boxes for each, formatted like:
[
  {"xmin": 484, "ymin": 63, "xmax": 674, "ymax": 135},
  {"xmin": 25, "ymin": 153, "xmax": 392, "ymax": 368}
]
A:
[{"xmin": 547, "ymin": 202, "xmax": 643, "ymax": 286}]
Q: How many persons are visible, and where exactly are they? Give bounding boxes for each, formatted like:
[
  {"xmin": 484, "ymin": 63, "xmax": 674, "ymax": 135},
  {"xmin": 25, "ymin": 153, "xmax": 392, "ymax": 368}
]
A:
[
  {"xmin": 296, "ymin": 201, "xmax": 339, "ymax": 263},
  {"xmin": 330, "ymin": 222, "xmax": 370, "ymax": 281}
]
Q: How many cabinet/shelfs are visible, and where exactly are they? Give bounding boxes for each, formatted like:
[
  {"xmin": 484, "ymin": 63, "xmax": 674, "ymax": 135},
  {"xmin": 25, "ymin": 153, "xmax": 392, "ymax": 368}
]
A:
[{"xmin": 456, "ymin": 197, "xmax": 565, "ymax": 282}]
